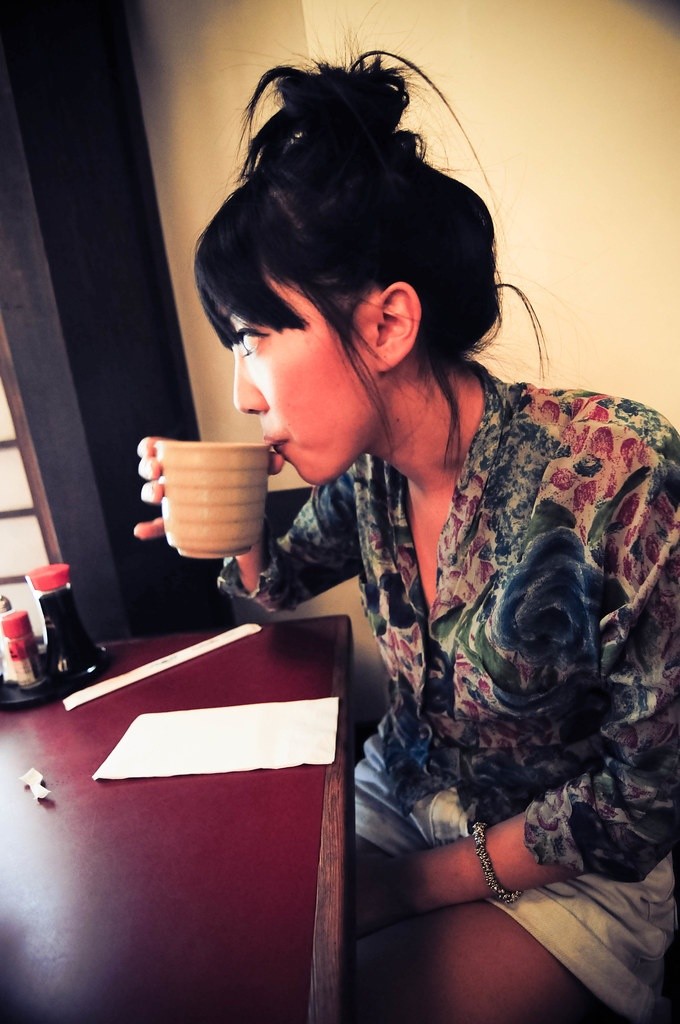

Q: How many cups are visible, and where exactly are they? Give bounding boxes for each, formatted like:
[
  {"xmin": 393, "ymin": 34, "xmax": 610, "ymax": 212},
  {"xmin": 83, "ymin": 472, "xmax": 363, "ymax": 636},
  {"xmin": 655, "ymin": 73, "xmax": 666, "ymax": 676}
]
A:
[{"xmin": 155, "ymin": 440, "xmax": 270, "ymax": 558}]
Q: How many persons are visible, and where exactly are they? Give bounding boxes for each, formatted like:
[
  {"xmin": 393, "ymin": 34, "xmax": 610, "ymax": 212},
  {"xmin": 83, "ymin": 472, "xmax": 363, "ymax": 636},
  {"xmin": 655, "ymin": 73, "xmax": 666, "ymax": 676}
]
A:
[{"xmin": 133, "ymin": 49, "xmax": 680, "ymax": 1024}]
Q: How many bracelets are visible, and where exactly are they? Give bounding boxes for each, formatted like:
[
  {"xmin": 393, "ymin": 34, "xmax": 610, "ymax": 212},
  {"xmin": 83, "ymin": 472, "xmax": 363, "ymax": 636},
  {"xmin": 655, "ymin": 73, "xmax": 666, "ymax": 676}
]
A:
[{"xmin": 472, "ymin": 822, "xmax": 524, "ymax": 904}]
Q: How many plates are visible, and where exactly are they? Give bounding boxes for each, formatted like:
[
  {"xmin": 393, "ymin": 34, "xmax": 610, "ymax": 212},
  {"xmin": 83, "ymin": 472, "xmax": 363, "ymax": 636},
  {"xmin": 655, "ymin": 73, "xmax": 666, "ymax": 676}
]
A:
[{"xmin": 0, "ymin": 645, "xmax": 106, "ymax": 710}]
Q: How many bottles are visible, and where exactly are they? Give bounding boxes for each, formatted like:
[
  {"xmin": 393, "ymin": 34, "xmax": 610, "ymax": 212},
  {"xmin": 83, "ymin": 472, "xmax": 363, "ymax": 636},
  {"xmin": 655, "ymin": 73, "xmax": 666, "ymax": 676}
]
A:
[
  {"xmin": 25, "ymin": 564, "xmax": 111, "ymax": 690},
  {"xmin": 0, "ymin": 595, "xmax": 47, "ymax": 691}
]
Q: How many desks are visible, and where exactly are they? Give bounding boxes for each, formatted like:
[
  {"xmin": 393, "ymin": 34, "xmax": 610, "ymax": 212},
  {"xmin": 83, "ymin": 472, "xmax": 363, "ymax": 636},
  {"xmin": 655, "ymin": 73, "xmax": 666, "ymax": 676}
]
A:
[{"xmin": 0, "ymin": 615, "xmax": 353, "ymax": 1024}]
